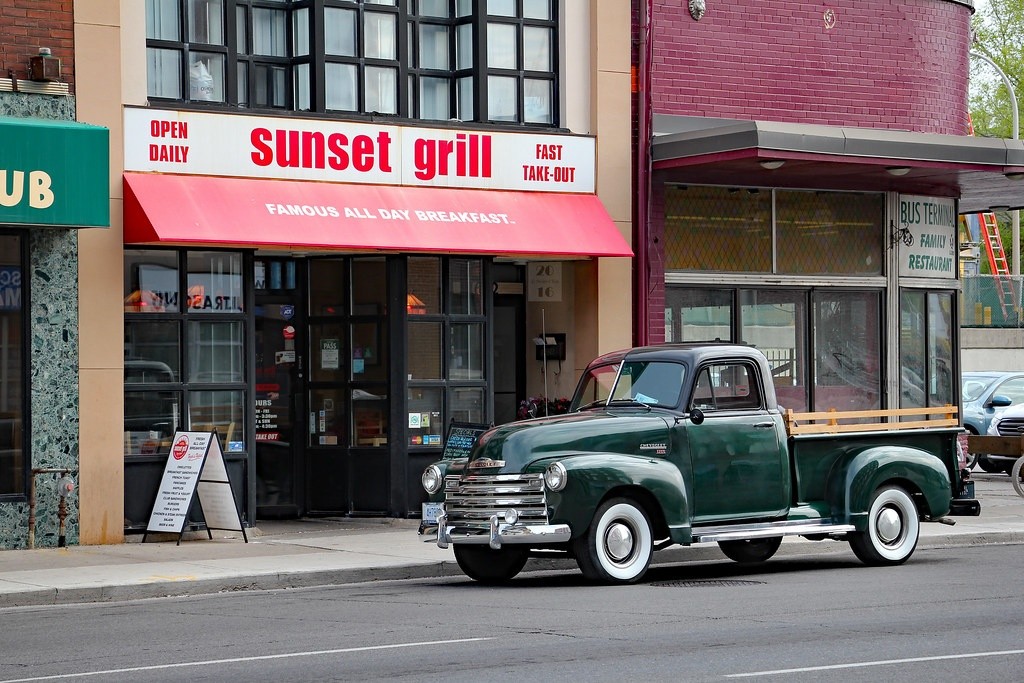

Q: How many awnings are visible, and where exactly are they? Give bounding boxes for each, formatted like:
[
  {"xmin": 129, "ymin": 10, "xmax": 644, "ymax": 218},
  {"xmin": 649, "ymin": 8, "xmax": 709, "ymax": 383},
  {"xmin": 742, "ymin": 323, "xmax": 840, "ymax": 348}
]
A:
[
  {"xmin": 121, "ymin": 172, "xmax": 639, "ymax": 256},
  {"xmin": 0, "ymin": 116, "xmax": 110, "ymax": 229}
]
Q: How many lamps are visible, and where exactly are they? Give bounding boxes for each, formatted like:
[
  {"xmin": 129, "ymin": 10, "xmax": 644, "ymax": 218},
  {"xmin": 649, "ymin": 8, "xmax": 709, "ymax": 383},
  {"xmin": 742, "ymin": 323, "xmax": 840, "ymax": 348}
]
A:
[
  {"xmin": 758, "ymin": 158, "xmax": 785, "ymax": 170},
  {"xmin": 884, "ymin": 166, "xmax": 913, "ymax": 176},
  {"xmin": 407, "ymin": 292, "xmax": 427, "ymax": 315},
  {"xmin": 1001, "ymin": 172, "xmax": 1024, "ymax": 181}
]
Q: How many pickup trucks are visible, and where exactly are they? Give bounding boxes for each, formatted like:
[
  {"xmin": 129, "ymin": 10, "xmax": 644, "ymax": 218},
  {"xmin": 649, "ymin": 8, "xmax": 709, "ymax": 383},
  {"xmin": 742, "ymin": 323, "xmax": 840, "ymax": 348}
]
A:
[{"xmin": 417, "ymin": 342, "xmax": 981, "ymax": 586}]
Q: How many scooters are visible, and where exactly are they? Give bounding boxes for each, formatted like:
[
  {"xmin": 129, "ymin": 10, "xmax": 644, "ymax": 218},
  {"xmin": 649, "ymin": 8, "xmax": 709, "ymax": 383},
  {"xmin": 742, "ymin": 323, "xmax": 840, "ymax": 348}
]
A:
[{"xmin": 1011, "ymin": 455, "xmax": 1024, "ymax": 498}]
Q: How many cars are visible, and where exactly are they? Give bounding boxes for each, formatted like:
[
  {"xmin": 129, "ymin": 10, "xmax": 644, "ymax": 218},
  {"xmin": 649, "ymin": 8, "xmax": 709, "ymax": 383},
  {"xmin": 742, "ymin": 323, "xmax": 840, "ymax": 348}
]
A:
[
  {"xmin": 962, "ymin": 370, "xmax": 1024, "ymax": 473},
  {"xmin": 985, "ymin": 402, "xmax": 1024, "ymax": 479}
]
